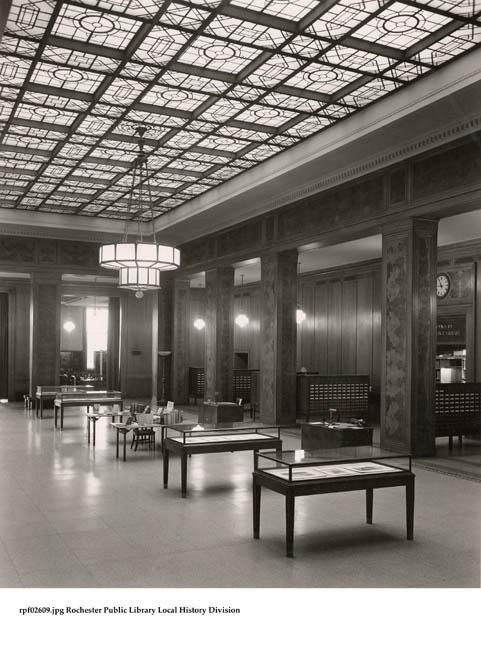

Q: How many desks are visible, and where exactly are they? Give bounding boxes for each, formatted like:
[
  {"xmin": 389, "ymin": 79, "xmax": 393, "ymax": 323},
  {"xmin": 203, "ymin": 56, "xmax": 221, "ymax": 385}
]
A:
[
  {"xmin": 302, "ymin": 421, "xmax": 374, "ymax": 451},
  {"xmin": 87, "ymin": 409, "xmax": 199, "ymax": 461},
  {"xmin": 198, "ymin": 401, "xmax": 244, "ymax": 429}
]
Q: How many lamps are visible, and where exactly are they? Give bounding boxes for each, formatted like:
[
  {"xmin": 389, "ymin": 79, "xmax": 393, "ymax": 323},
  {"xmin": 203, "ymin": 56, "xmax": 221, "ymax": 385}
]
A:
[
  {"xmin": 98, "ymin": 126, "xmax": 182, "ymax": 300},
  {"xmin": 192, "ymin": 261, "xmax": 306, "ymax": 330}
]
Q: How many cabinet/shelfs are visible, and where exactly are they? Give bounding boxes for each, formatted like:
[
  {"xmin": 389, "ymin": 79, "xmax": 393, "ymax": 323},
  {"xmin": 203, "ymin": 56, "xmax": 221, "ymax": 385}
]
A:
[
  {"xmin": 188, "ymin": 367, "xmax": 260, "ymax": 403},
  {"xmin": 295, "ymin": 373, "xmax": 370, "ymax": 422},
  {"xmin": 435, "ymin": 383, "xmax": 481, "ymax": 450}
]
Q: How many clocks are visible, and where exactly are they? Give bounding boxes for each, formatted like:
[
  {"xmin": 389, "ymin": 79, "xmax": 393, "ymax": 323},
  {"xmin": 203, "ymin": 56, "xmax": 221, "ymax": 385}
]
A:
[{"xmin": 436, "ymin": 273, "xmax": 451, "ymax": 300}]
